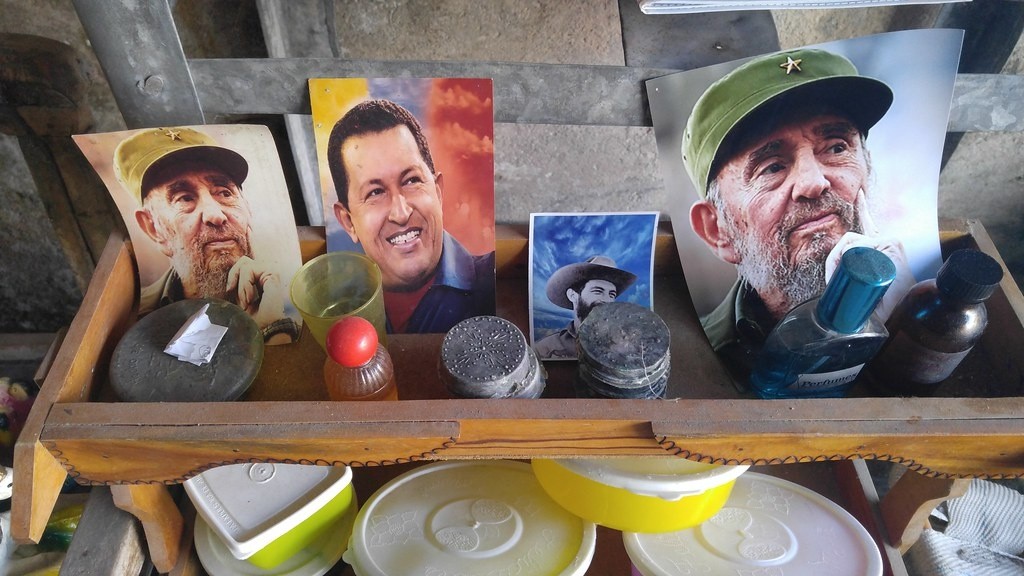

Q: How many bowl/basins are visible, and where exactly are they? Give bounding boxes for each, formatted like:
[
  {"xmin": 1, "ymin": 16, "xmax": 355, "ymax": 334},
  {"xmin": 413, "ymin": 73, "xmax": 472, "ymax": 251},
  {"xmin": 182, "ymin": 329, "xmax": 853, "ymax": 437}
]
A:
[{"xmin": 183, "ymin": 456, "xmax": 883, "ymax": 576}]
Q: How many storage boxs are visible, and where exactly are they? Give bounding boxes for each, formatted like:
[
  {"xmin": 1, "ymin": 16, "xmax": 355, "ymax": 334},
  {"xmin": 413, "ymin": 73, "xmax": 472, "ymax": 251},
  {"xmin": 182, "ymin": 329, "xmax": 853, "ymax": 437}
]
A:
[{"xmin": 183, "ymin": 464, "xmax": 358, "ymax": 571}]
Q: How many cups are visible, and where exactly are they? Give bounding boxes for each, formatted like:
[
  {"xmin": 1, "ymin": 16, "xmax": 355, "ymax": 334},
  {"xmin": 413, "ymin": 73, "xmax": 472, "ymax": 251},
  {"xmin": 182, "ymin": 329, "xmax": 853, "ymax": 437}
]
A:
[{"xmin": 291, "ymin": 251, "xmax": 389, "ymax": 356}]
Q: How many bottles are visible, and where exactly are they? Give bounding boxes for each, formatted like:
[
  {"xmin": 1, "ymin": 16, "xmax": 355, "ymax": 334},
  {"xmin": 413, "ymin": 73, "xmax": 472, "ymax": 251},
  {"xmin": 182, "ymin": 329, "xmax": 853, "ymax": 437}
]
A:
[
  {"xmin": 321, "ymin": 316, "xmax": 398, "ymax": 401},
  {"xmin": 867, "ymin": 249, "xmax": 1003, "ymax": 397},
  {"xmin": 746, "ymin": 247, "xmax": 897, "ymax": 399}
]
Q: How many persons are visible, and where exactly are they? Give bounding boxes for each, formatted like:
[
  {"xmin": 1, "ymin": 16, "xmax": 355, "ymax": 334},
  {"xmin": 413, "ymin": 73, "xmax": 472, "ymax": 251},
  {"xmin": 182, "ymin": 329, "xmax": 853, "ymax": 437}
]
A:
[
  {"xmin": 327, "ymin": 100, "xmax": 497, "ymax": 333},
  {"xmin": 113, "ymin": 127, "xmax": 298, "ymax": 346},
  {"xmin": 534, "ymin": 255, "xmax": 637, "ymax": 361},
  {"xmin": 681, "ymin": 49, "xmax": 918, "ymax": 398}
]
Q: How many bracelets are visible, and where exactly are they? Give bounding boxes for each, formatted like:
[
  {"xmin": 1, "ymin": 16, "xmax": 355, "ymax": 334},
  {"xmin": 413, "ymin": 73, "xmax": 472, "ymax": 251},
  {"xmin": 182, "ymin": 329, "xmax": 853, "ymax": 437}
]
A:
[{"xmin": 262, "ymin": 317, "xmax": 298, "ymax": 342}]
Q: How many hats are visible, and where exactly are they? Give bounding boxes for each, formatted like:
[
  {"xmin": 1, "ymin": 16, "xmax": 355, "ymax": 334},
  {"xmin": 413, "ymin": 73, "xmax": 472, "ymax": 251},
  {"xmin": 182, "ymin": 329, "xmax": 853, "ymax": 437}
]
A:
[
  {"xmin": 546, "ymin": 256, "xmax": 637, "ymax": 310},
  {"xmin": 113, "ymin": 128, "xmax": 248, "ymax": 207},
  {"xmin": 681, "ymin": 49, "xmax": 894, "ymax": 199}
]
